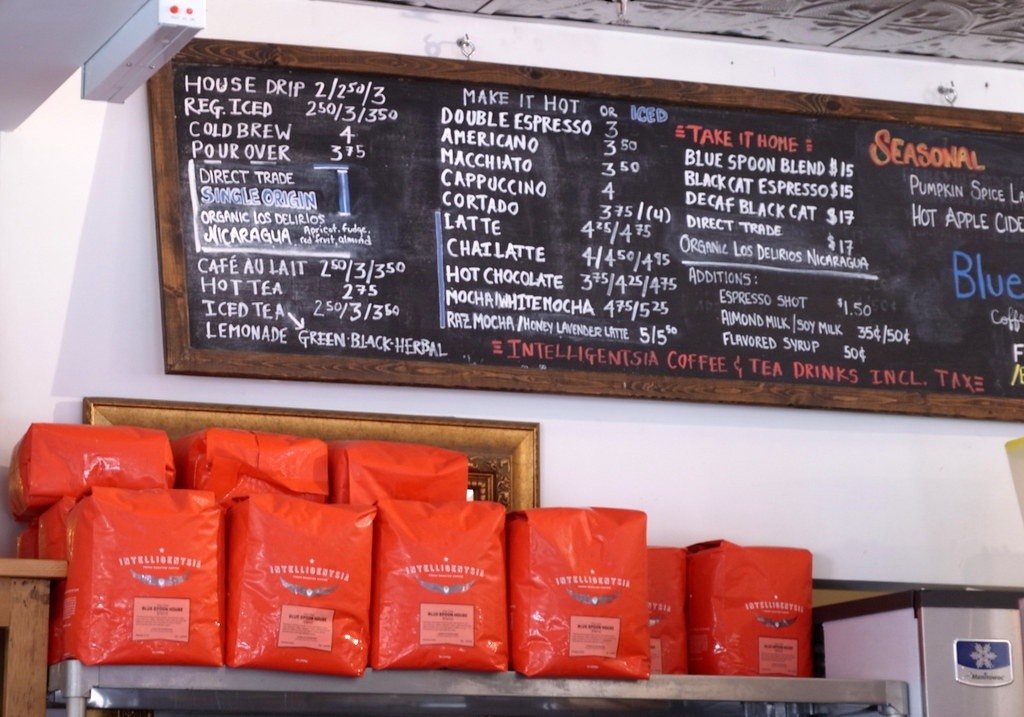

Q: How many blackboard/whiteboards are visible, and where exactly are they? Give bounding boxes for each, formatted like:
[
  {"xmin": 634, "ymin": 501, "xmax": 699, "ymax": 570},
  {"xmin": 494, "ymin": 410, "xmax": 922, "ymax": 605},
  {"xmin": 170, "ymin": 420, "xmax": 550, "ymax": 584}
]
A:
[{"xmin": 148, "ymin": 37, "xmax": 1024, "ymax": 425}]
